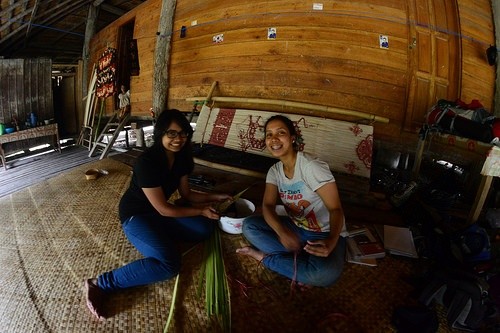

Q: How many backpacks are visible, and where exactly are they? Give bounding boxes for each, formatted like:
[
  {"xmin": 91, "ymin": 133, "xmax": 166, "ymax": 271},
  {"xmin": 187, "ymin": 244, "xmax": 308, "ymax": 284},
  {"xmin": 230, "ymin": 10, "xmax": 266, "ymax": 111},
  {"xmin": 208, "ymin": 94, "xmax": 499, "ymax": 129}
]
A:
[{"xmin": 445, "ymin": 283, "xmax": 487, "ymax": 332}]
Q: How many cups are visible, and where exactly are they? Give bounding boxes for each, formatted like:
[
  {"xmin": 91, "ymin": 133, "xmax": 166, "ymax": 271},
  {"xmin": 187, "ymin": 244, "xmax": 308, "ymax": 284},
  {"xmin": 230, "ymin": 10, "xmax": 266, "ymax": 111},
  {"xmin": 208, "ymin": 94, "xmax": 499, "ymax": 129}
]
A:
[{"xmin": 44, "ymin": 120, "xmax": 50, "ymax": 125}]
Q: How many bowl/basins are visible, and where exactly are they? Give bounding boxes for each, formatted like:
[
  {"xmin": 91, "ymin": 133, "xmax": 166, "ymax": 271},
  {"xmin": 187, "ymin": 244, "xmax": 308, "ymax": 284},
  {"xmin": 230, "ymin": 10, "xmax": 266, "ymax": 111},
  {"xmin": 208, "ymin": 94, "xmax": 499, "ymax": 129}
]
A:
[
  {"xmin": 85, "ymin": 168, "xmax": 99, "ymax": 180},
  {"xmin": 5, "ymin": 128, "xmax": 15, "ymax": 134},
  {"xmin": 213, "ymin": 197, "xmax": 257, "ymax": 235}
]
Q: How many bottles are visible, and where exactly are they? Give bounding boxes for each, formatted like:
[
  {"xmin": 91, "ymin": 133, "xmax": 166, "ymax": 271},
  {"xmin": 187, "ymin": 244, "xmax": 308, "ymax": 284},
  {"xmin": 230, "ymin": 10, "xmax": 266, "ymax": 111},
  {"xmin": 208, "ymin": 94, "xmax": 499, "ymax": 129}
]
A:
[
  {"xmin": 12, "ymin": 113, "xmax": 37, "ymax": 132},
  {"xmin": 0, "ymin": 123, "xmax": 4, "ymax": 135}
]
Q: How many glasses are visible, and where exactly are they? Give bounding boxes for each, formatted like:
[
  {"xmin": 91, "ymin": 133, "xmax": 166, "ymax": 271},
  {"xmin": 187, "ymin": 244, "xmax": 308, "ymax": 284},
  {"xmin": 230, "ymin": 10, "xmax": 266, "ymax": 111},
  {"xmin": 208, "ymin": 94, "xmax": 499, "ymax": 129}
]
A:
[{"xmin": 166, "ymin": 130, "xmax": 187, "ymax": 139}]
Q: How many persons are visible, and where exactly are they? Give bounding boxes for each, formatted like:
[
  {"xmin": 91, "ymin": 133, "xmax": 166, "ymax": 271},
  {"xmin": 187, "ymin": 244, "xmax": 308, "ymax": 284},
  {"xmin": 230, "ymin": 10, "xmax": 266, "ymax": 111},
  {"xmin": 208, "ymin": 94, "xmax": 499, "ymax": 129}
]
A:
[
  {"xmin": 118, "ymin": 85, "xmax": 131, "ymax": 118},
  {"xmin": 85, "ymin": 109, "xmax": 227, "ymax": 322},
  {"xmin": 235, "ymin": 116, "xmax": 344, "ymax": 287}
]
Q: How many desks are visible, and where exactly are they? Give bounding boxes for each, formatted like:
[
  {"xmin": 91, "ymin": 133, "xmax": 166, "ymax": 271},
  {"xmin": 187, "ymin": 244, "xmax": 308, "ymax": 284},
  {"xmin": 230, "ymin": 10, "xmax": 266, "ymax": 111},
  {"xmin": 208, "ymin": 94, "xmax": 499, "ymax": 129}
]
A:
[
  {"xmin": 0, "ymin": 123, "xmax": 62, "ymax": 171},
  {"xmin": 411, "ymin": 123, "xmax": 500, "ymax": 229}
]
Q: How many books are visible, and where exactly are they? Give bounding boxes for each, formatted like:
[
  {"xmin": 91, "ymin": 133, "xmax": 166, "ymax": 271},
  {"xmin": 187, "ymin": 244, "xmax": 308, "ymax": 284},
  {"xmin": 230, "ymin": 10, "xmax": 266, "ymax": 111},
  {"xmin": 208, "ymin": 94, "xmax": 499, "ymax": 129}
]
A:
[{"xmin": 339, "ymin": 223, "xmax": 418, "ymax": 266}]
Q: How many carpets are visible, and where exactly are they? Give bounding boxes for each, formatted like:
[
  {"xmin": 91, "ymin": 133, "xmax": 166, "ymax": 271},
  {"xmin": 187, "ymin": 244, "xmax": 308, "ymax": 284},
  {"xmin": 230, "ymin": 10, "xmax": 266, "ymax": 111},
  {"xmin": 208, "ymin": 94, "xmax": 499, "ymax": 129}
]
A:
[{"xmin": 0, "ymin": 158, "xmax": 449, "ymax": 333}]
